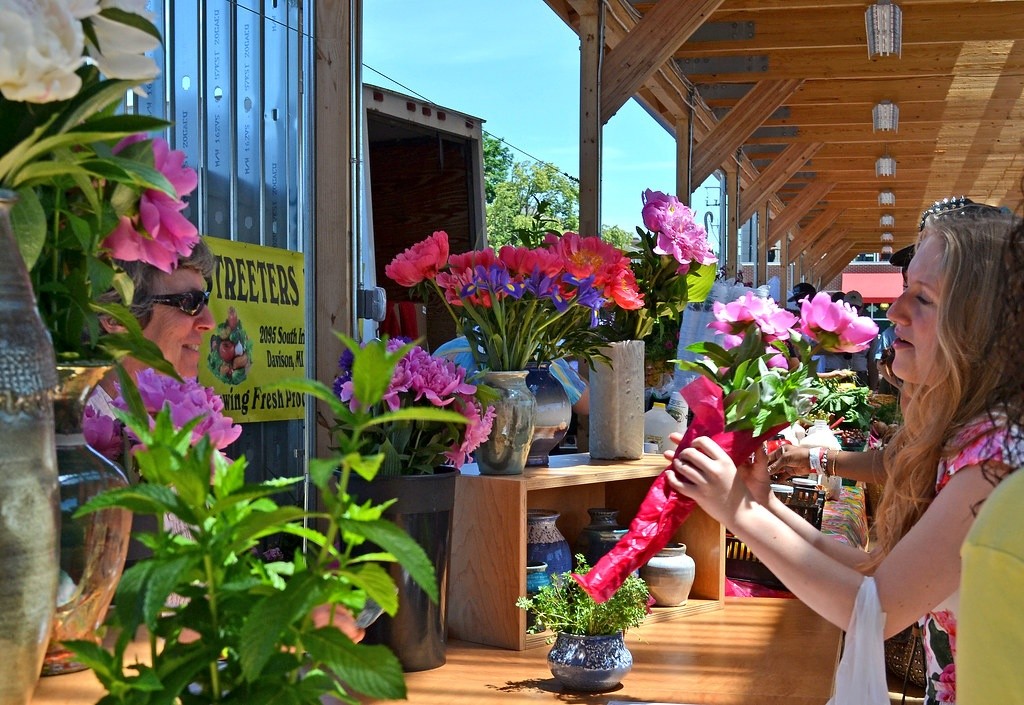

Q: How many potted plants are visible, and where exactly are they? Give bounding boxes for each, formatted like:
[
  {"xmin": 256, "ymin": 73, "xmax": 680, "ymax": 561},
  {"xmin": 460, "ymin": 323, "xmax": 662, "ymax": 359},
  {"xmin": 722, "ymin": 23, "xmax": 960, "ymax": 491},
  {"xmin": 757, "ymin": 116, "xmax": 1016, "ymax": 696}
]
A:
[{"xmin": 515, "ymin": 552, "xmax": 650, "ymax": 692}]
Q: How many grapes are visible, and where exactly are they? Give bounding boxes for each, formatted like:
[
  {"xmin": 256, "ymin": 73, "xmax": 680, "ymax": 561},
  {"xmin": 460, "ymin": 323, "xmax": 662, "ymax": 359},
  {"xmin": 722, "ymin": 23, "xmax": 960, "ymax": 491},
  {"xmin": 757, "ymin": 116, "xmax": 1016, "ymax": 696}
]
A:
[{"xmin": 841, "ymin": 429, "xmax": 868, "ymax": 447}]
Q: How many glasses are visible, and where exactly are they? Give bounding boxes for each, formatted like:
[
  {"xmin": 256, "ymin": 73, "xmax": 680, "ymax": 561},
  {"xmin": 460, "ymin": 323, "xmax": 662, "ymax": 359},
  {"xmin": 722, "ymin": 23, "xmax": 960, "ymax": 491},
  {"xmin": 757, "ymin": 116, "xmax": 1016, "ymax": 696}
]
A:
[
  {"xmin": 795, "ymin": 303, "xmax": 801, "ymax": 307},
  {"xmin": 143, "ymin": 289, "xmax": 210, "ymax": 316}
]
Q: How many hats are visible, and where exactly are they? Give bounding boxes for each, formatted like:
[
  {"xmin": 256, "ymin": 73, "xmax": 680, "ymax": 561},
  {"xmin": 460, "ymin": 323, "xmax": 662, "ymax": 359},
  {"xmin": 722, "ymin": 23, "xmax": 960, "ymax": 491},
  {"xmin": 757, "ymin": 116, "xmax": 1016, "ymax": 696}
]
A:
[
  {"xmin": 788, "ymin": 283, "xmax": 816, "ymax": 302},
  {"xmin": 844, "ymin": 291, "xmax": 862, "ymax": 307},
  {"xmin": 888, "ymin": 196, "xmax": 1010, "ymax": 266}
]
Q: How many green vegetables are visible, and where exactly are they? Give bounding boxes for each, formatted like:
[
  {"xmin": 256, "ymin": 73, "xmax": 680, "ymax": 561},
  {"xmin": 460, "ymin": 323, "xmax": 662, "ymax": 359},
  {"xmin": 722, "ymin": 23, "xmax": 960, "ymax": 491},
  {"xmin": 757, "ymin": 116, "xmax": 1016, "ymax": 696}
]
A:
[{"xmin": 206, "ymin": 320, "xmax": 253, "ymax": 384}]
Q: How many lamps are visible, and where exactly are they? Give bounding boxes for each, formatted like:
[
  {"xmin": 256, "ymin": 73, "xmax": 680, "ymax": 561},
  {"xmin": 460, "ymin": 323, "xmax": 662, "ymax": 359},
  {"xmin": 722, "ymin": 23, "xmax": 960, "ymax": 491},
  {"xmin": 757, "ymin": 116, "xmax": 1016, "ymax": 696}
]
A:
[
  {"xmin": 865, "ymin": 0, "xmax": 903, "ymax": 62},
  {"xmin": 882, "ymin": 245, "xmax": 893, "ymax": 253},
  {"xmin": 880, "ymin": 303, "xmax": 889, "ymax": 311},
  {"xmin": 766, "ymin": 432, "xmax": 793, "ymax": 457},
  {"xmin": 870, "ymin": 100, "xmax": 900, "ymax": 134},
  {"xmin": 879, "ymin": 214, "xmax": 895, "ymax": 227},
  {"xmin": 878, "ymin": 190, "xmax": 896, "ymax": 208},
  {"xmin": 786, "ymin": 421, "xmax": 805, "ymax": 439},
  {"xmin": 875, "ymin": 153, "xmax": 897, "ymax": 179},
  {"xmin": 799, "ymin": 420, "xmax": 845, "ymax": 502},
  {"xmin": 880, "ymin": 232, "xmax": 894, "ymax": 242}
]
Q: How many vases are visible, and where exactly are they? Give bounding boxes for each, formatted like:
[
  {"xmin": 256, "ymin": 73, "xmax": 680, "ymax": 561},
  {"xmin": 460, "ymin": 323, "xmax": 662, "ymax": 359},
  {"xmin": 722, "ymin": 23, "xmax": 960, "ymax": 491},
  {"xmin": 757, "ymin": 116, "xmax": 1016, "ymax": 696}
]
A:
[
  {"xmin": 521, "ymin": 363, "xmax": 571, "ymax": 468},
  {"xmin": 584, "ymin": 340, "xmax": 645, "ymax": 462},
  {"xmin": 337, "ymin": 460, "xmax": 459, "ymax": 673},
  {"xmin": 453, "ymin": 370, "xmax": 536, "ymax": 480},
  {"xmin": 39, "ymin": 360, "xmax": 138, "ymax": 675},
  {"xmin": 525, "ymin": 508, "xmax": 697, "ymax": 621},
  {"xmin": 1, "ymin": 186, "xmax": 71, "ymax": 704}
]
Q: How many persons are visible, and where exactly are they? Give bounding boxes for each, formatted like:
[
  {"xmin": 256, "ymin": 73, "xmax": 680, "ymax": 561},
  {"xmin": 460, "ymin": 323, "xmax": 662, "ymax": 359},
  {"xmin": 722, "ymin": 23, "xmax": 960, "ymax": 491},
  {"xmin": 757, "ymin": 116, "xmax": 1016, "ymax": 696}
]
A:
[
  {"xmin": 661, "ymin": 200, "xmax": 1024, "ymax": 705},
  {"xmin": 60, "ymin": 236, "xmax": 365, "ymax": 662},
  {"xmin": 428, "ymin": 337, "xmax": 591, "ymax": 418}
]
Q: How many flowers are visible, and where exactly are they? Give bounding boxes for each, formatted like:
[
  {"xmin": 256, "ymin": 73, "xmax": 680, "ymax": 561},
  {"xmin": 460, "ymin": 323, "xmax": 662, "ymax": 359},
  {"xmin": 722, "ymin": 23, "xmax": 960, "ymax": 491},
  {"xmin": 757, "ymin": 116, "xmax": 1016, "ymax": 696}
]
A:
[{"xmin": 1, "ymin": 0, "xmax": 862, "ymax": 635}]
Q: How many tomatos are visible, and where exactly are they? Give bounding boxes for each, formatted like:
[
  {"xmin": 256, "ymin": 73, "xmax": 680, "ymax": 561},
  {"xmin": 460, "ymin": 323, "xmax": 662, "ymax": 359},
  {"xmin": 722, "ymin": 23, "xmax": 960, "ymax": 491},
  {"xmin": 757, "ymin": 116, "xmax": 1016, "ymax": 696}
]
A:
[{"xmin": 213, "ymin": 307, "xmax": 247, "ymax": 378}]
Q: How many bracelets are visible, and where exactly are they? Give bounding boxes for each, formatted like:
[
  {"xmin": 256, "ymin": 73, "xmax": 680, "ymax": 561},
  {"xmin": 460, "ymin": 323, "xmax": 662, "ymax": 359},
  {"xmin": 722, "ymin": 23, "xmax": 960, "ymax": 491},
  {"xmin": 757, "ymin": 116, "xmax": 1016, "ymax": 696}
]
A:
[
  {"xmin": 821, "ymin": 448, "xmax": 830, "ymax": 474},
  {"xmin": 808, "ymin": 447, "xmax": 822, "ymax": 475},
  {"xmin": 832, "ymin": 450, "xmax": 841, "ymax": 475}
]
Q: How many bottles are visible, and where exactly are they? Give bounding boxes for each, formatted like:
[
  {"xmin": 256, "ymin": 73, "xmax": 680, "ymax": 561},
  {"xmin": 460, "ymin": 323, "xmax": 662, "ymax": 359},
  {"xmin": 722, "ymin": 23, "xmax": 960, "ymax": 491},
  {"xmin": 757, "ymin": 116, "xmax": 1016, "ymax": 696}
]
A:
[
  {"xmin": 527, "ymin": 507, "xmax": 695, "ymax": 608},
  {"xmin": 644, "ymin": 402, "xmax": 680, "ymax": 455},
  {"xmin": 767, "ymin": 418, "xmax": 842, "ymax": 501}
]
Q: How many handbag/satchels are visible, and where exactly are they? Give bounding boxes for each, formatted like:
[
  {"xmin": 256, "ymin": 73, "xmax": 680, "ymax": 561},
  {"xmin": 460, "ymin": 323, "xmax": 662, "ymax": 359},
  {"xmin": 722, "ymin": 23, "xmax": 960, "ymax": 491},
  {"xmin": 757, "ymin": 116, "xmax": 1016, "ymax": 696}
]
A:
[
  {"xmin": 881, "ymin": 616, "xmax": 926, "ymax": 688},
  {"xmin": 825, "ymin": 575, "xmax": 891, "ymax": 705}
]
